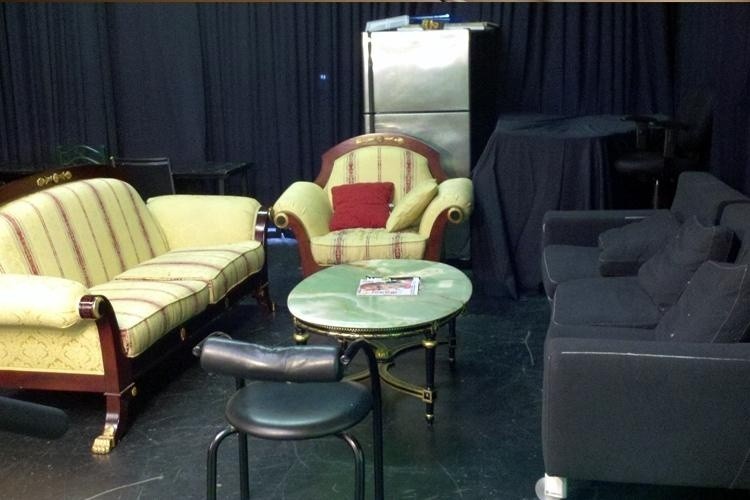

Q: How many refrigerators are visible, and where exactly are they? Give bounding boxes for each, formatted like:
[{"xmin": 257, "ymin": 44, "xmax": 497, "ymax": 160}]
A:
[{"xmin": 362, "ymin": 28, "xmax": 500, "ymax": 269}]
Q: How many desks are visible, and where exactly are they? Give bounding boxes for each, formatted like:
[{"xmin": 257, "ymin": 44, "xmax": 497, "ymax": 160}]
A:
[
  {"xmin": 1, "ymin": 160, "xmax": 253, "ymax": 194},
  {"xmin": 490, "ymin": 108, "xmax": 642, "ymax": 299}
]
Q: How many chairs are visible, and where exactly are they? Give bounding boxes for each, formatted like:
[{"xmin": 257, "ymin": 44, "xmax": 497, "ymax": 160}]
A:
[
  {"xmin": 109, "ymin": 157, "xmax": 177, "ymax": 201},
  {"xmin": 617, "ymin": 108, "xmax": 724, "ymax": 209},
  {"xmin": 187, "ymin": 326, "xmax": 387, "ymax": 500},
  {"xmin": 50, "ymin": 140, "xmax": 110, "ymax": 170}
]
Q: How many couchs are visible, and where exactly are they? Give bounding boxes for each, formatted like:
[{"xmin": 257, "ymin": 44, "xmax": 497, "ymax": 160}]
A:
[
  {"xmin": 534, "ymin": 166, "xmax": 749, "ymax": 500},
  {"xmin": 268, "ymin": 131, "xmax": 472, "ymax": 275},
  {"xmin": 0, "ymin": 167, "xmax": 271, "ymax": 459}
]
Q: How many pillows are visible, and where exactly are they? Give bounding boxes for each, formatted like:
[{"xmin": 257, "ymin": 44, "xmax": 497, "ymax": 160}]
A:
[
  {"xmin": 328, "ymin": 181, "xmax": 394, "ymax": 232},
  {"xmin": 652, "ymin": 254, "xmax": 749, "ymax": 345},
  {"xmin": 635, "ymin": 214, "xmax": 734, "ymax": 309},
  {"xmin": 383, "ymin": 176, "xmax": 440, "ymax": 232},
  {"xmin": 594, "ymin": 205, "xmax": 687, "ymax": 274}
]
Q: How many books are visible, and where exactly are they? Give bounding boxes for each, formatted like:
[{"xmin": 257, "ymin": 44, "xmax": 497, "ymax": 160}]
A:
[{"xmin": 357, "ymin": 274, "xmax": 421, "ymax": 297}]
